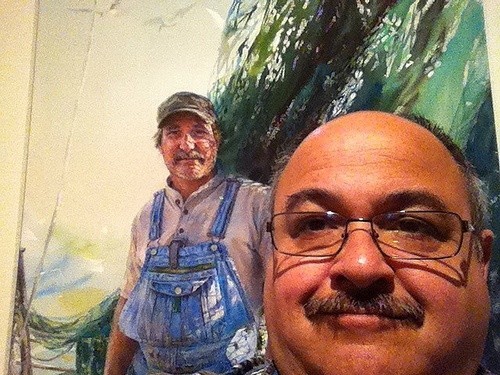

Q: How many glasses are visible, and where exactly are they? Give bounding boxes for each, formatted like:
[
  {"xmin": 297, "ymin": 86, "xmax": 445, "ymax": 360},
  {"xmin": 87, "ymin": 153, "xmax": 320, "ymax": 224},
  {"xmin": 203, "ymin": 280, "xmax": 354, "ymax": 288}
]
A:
[{"xmin": 266, "ymin": 211, "xmax": 481, "ymax": 260}]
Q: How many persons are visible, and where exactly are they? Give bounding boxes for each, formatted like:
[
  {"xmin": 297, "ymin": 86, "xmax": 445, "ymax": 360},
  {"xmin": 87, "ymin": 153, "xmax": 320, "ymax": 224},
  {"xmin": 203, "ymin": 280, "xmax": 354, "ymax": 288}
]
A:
[
  {"xmin": 262, "ymin": 110, "xmax": 495, "ymax": 375},
  {"xmin": 104, "ymin": 91, "xmax": 279, "ymax": 375}
]
